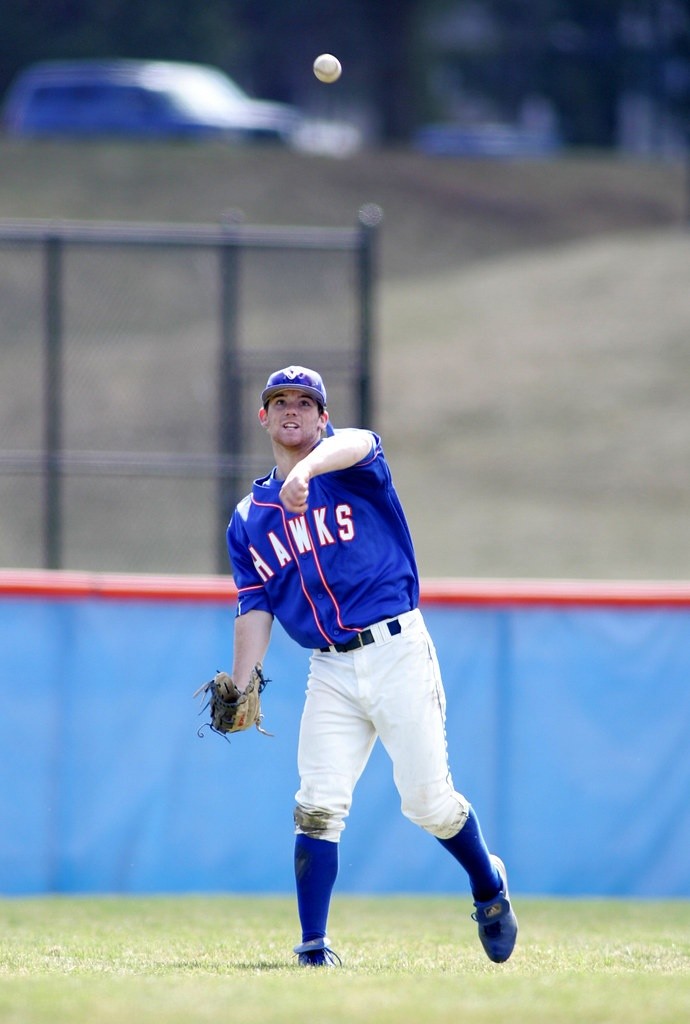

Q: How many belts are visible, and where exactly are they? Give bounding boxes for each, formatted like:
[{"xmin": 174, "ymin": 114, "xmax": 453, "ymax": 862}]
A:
[{"xmin": 319, "ymin": 620, "xmax": 402, "ymax": 653}]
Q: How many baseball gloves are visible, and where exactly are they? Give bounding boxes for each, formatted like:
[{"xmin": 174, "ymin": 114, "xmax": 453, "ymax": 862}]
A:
[{"xmin": 197, "ymin": 657, "xmax": 276, "ymax": 745}]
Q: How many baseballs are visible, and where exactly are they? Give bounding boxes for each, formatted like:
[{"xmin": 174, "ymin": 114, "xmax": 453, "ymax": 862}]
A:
[{"xmin": 312, "ymin": 53, "xmax": 343, "ymax": 85}]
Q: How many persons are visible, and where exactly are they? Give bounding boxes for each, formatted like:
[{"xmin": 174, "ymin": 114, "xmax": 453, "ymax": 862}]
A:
[{"xmin": 220, "ymin": 366, "xmax": 517, "ymax": 966}]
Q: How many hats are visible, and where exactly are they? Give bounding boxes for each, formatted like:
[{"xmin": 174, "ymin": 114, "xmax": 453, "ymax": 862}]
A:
[{"xmin": 261, "ymin": 364, "xmax": 328, "ymax": 411}]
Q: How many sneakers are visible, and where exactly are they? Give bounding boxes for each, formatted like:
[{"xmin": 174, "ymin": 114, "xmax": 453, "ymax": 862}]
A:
[
  {"xmin": 471, "ymin": 855, "xmax": 518, "ymax": 964},
  {"xmin": 294, "ymin": 938, "xmax": 342, "ymax": 969}
]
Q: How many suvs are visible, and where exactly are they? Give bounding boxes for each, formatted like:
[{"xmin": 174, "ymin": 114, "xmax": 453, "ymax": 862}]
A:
[{"xmin": 4, "ymin": 57, "xmax": 357, "ymax": 158}]
